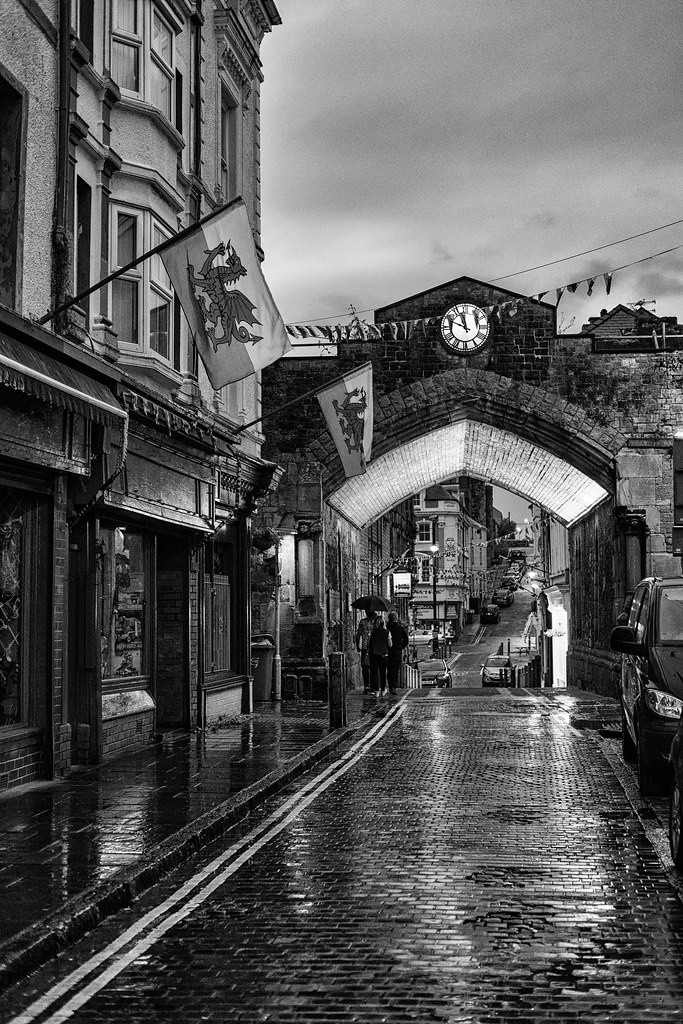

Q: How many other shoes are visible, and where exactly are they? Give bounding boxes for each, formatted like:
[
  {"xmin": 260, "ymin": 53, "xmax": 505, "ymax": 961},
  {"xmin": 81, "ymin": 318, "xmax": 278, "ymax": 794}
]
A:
[
  {"xmin": 381, "ymin": 688, "xmax": 388, "ymax": 696},
  {"xmin": 390, "ymin": 687, "xmax": 398, "ymax": 695},
  {"xmin": 375, "ymin": 691, "xmax": 380, "ymax": 698}
]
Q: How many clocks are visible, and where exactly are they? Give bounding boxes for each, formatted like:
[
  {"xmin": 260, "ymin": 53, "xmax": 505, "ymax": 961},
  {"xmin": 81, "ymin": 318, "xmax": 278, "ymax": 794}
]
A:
[{"xmin": 437, "ymin": 299, "xmax": 495, "ymax": 356}]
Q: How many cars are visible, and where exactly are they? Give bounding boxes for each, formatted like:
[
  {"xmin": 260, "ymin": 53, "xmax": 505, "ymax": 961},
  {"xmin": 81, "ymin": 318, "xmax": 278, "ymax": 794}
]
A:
[
  {"xmin": 500, "ymin": 563, "xmax": 527, "ymax": 590},
  {"xmin": 492, "ymin": 589, "xmax": 514, "ymax": 606},
  {"xmin": 414, "ymin": 659, "xmax": 452, "ymax": 688}
]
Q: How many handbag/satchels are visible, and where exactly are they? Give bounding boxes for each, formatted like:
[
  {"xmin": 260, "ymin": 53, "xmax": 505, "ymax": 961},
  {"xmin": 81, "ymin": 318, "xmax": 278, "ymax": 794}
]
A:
[{"xmin": 363, "ymin": 652, "xmax": 370, "ymax": 669}]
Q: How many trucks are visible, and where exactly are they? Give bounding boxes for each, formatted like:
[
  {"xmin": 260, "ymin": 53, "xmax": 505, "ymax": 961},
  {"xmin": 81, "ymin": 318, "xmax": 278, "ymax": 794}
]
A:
[{"xmin": 510, "ymin": 550, "xmax": 528, "ymax": 565}]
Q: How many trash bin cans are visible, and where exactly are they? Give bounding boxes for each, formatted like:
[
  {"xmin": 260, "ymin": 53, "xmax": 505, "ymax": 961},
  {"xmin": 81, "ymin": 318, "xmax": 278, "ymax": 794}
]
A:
[{"xmin": 250, "ymin": 634, "xmax": 275, "ymax": 702}]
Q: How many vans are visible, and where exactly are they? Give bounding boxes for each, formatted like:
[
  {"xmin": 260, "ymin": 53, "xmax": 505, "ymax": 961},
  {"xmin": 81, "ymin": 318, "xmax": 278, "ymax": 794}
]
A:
[{"xmin": 610, "ymin": 576, "xmax": 683, "ymax": 870}]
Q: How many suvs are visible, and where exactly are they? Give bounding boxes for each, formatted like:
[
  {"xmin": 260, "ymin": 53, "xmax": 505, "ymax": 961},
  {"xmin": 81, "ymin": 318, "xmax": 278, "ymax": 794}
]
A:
[
  {"xmin": 479, "ymin": 655, "xmax": 517, "ymax": 687},
  {"xmin": 480, "ymin": 604, "xmax": 501, "ymax": 624}
]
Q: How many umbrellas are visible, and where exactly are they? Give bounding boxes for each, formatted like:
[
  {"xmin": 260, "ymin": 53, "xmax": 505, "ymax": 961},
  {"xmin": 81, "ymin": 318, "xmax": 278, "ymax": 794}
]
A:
[{"xmin": 350, "ymin": 595, "xmax": 395, "ymax": 612}]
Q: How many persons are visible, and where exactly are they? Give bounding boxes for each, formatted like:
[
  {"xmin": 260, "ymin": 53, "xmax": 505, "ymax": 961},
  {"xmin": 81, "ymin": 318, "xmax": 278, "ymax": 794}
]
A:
[
  {"xmin": 357, "ymin": 609, "xmax": 377, "ymax": 694},
  {"xmin": 387, "ymin": 612, "xmax": 409, "ymax": 688},
  {"xmin": 368, "ymin": 617, "xmax": 392, "ymax": 698}
]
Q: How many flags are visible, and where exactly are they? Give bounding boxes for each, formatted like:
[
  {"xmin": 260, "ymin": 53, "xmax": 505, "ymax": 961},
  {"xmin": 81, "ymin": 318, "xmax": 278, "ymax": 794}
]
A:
[
  {"xmin": 154, "ymin": 198, "xmax": 293, "ymax": 391},
  {"xmin": 315, "ymin": 361, "xmax": 375, "ymax": 478},
  {"xmin": 287, "ymin": 271, "xmax": 613, "ymax": 339}
]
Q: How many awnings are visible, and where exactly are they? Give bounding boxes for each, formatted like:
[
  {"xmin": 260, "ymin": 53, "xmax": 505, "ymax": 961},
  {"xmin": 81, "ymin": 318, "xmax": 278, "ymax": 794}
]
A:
[
  {"xmin": 1, "ymin": 334, "xmax": 130, "ymax": 430},
  {"xmin": 102, "ymin": 493, "xmax": 216, "ymax": 534}
]
